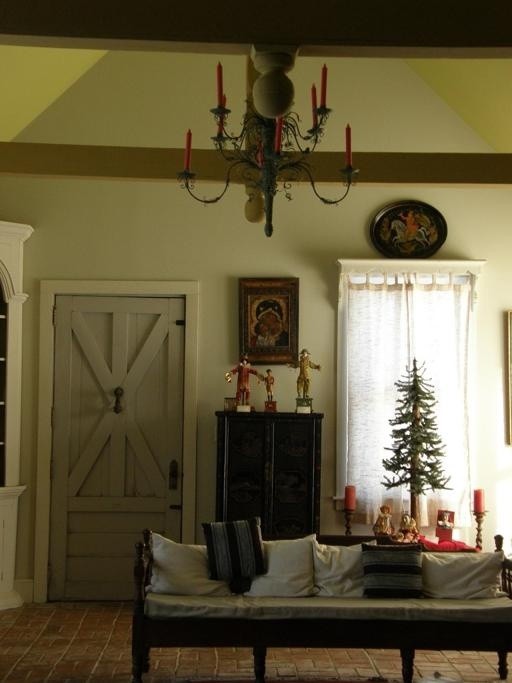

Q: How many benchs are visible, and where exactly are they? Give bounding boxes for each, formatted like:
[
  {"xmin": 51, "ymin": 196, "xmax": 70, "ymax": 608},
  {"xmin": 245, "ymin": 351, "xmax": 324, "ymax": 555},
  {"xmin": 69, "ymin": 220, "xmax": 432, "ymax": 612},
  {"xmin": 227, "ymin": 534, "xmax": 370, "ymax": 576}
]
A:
[{"xmin": 132, "ymin": 527, "xmax": 512, "ymax": 683}]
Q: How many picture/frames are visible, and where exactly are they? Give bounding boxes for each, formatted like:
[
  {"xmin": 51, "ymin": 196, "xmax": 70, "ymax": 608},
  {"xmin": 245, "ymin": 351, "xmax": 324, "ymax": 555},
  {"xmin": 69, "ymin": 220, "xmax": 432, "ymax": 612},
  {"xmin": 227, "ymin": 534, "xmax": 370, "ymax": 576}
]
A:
[{"xmin": 236, "ymin": 275, "xmax": 301, "ymax": 367}]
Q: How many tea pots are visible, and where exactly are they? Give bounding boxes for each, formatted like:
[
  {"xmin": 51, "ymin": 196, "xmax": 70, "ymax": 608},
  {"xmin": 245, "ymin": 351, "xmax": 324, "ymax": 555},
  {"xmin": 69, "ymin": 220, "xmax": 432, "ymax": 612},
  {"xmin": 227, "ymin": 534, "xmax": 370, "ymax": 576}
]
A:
[{"xmin": 371, "ymin": 511, "xmax": 393, "ymax": 536}]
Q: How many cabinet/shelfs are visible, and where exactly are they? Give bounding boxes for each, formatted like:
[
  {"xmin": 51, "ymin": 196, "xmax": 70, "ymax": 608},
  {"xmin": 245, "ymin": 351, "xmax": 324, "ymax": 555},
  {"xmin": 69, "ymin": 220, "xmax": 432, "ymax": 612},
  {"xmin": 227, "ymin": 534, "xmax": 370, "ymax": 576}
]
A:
[{"xmin": 211, "ymin": 408, "xmax": 325, "ymax": 540}]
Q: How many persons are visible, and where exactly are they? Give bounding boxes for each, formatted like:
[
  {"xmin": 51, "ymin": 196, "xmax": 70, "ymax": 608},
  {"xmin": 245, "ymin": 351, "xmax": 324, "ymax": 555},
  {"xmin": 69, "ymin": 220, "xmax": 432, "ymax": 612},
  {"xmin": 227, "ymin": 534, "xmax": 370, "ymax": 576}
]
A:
[
  {"xmin": 372, "ymin": 504, "xmax": 392, "ymax": 532},
  {"xmin": 264, "ymin": 368, "xmax": 275, "ymax": 400},
  {"xmin": 228, "ymin": 355, "xmax": 264, "ymax": 405},
  {"xmin": 435, "ymin": 511, "xmax": 454, "ymax": 529},
  {"xmin": 395, "ymin": 511, "xmax": 417, "ymax": 542},
  {"xmin": 286, "ymin": 348, "xmax": 320, "ymax": 398}
]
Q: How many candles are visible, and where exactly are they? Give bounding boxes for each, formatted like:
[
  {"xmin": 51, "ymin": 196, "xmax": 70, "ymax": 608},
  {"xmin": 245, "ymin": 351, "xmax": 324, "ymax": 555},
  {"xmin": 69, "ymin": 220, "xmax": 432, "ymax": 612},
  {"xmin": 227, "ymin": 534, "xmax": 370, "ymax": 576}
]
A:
[
  {"xmin": 181, "ymin": 58, "xmax": 352, "ymax": 169},
  {"xmin": 344, "ymin": 485, "xmax": 356, "ymax": 510},
  {"xmin": 474, "ymin": 489, "xmax": 485, "ymax": 512}
]
